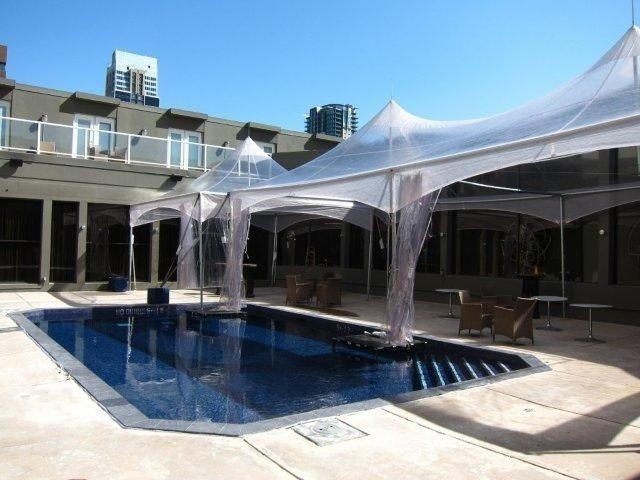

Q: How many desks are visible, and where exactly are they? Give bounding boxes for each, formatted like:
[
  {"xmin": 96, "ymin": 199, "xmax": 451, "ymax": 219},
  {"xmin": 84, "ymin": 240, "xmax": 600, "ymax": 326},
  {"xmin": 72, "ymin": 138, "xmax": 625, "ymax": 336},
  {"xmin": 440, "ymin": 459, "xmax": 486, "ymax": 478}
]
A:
[
  {"xmin": 434, "ymin": 287, "xmax": 463, "ymax": 318},
  {"xmin": 533, "ymin": 295, "xmax": 613, "ymax": 344}
]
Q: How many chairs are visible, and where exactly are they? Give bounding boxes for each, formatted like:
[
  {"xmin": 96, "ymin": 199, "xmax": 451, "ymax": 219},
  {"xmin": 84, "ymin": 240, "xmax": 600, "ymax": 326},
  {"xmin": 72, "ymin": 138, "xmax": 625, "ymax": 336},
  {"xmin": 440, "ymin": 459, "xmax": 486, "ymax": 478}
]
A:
[
  {"xmin": 286, "ymin": 271, "xmax": 344, "ymax": 310},
  {"xmin": 456, "ymin": 289, "xmax": 540, "ymax": 347},
  {"xmin": 91, "ymin": 144, "xmax": 129, "ymax": 164}
]
